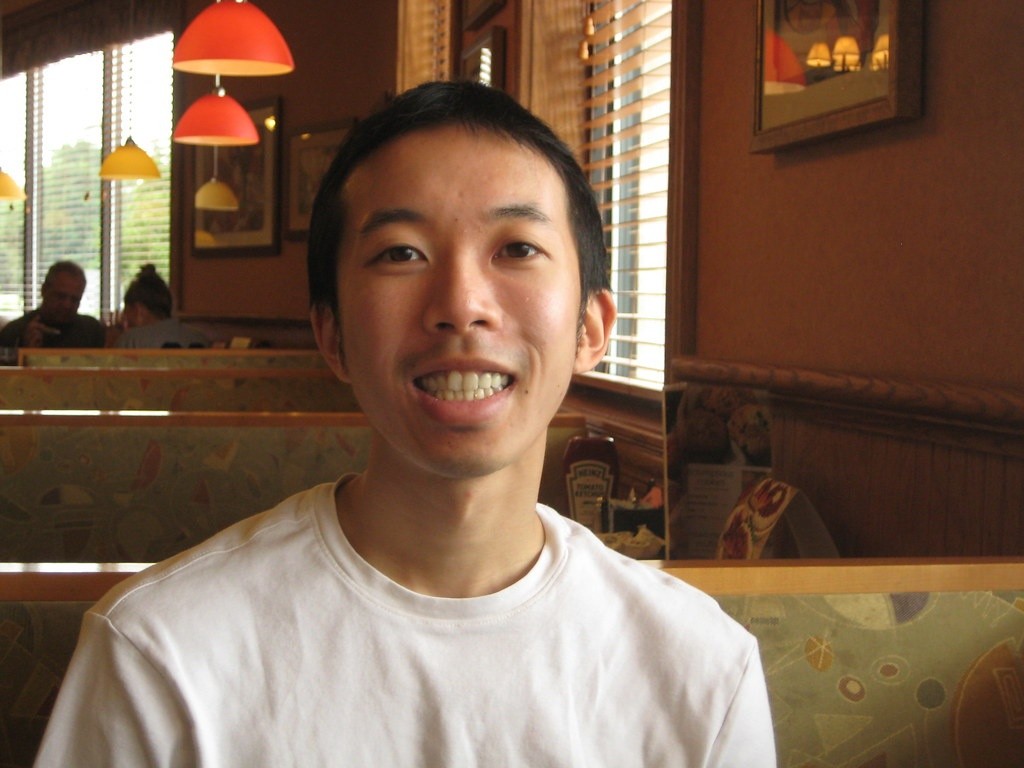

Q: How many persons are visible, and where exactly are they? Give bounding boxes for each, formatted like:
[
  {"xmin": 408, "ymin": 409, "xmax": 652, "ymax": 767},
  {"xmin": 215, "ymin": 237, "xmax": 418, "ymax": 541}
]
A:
[
  {"xmin": 30, "ymin": 80, "xmax": 778, "ymax": 768},
  {"xmin": 0, "ymin": 262, "xmax": 107, "ymax": 349},
  {"xmin": 110, "ymin": 264, "xmax": 211, "ymax": 348}
]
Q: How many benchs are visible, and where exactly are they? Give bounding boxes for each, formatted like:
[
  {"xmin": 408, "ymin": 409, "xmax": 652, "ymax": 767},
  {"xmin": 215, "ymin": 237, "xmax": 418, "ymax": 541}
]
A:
[
  {"xmin": 16, "ymin": 347, "xmax": 326, "ymax": 368},
  {"xmin": 0, "ymin": 407, "xmax": 588, "ymax": 564},
  {"xmin": 0, "ymin": 365, "xmax": 363, "ymax": 410},
  {"xmin": 1, "ymin": 555, "xmax": 1024, "ymax": 768}
]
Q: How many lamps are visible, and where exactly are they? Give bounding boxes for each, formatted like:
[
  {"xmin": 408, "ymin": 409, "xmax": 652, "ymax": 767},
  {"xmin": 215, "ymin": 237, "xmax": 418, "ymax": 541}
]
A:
[
  {"xmin": 175, "ymin": 0, "xmax": 295, "ymax": 76},
  {"xmin": 98, "ymin": 0, "xmax": 162, "ymax": 181},
  {"xmin": 194, "ymin": 147, "xmax": 238, "ymax": 212},
  {"xmin": 0, "ymin": 164, "xmax": 28, "ymax": 201},
  {"xmin": 173, "ymin": 74, "xmax": 260, "ymax": 146},
  {"xmin": 763, "ymin": 24, "xmax": 890, "ymax": 97}
]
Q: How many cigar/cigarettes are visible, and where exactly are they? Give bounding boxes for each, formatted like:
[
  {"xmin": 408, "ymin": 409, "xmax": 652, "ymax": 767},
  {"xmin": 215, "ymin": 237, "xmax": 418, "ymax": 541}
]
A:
[{"xmin": 42, "ymin": 324, "xmax": 61, "ymax": 336}]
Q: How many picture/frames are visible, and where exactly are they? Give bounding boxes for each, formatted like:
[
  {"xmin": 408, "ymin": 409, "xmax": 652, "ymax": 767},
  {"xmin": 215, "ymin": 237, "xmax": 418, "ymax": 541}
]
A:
[
  {"xmin": 746, "ymin": 1, "xmax": 926, "ymax": 156},
  {"xmin": 190, "ymin": 92, "xmax": 285, "ymax": 260},
  {"xmin": 279, "ymin": 117, "xmax": 361, "ymax": 242}
]
